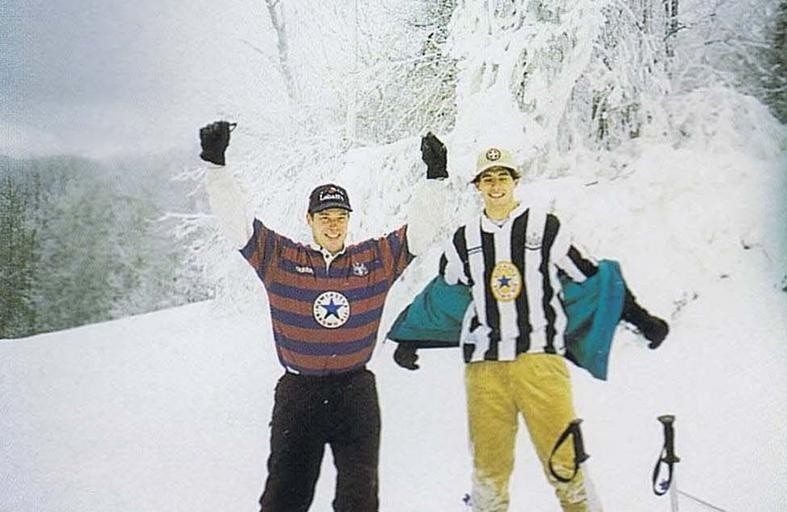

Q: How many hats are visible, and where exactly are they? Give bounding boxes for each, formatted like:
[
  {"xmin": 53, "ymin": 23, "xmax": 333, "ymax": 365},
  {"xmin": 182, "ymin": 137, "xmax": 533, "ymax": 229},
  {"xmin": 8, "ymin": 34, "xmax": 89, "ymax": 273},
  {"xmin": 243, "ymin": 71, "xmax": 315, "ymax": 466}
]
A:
[
  {"xmin": 308, "ymin": 183, "xmax": 353, "ymax": 214},
  {"xmin": 467, "ymin": 147, "xmax": 522, "ymax": 185}
]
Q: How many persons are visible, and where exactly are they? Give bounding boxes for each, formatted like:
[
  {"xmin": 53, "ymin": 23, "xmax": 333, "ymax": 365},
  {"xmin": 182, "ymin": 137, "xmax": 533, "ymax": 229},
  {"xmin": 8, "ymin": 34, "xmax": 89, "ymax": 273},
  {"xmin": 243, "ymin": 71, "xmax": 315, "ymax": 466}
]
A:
[
  {"xmin": 198, "ymin": 121, "xmax": 449, "ymax": 512},
  {"xmin": 383, "ymin": 144, "xmax": 668, "ymax": 512}
]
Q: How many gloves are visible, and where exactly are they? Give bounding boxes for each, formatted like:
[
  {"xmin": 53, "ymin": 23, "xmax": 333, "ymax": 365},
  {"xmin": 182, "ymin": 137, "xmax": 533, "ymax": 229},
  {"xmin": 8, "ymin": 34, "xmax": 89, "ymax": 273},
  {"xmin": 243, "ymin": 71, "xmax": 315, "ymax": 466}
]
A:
[
  {"xmin": 196, "ymin": 119, "xmax": 233, "ymax": 165},
  {"xmin": 419, "ymin": 130, "xmax": 450, "ymax": 181},
  {"xmin": 391, "ymin": 342, "xmax": 422, "ymax": 370},
  {"xmin": 638, "ymin": 314, "xmax": 669, "ymax": 351}
]
